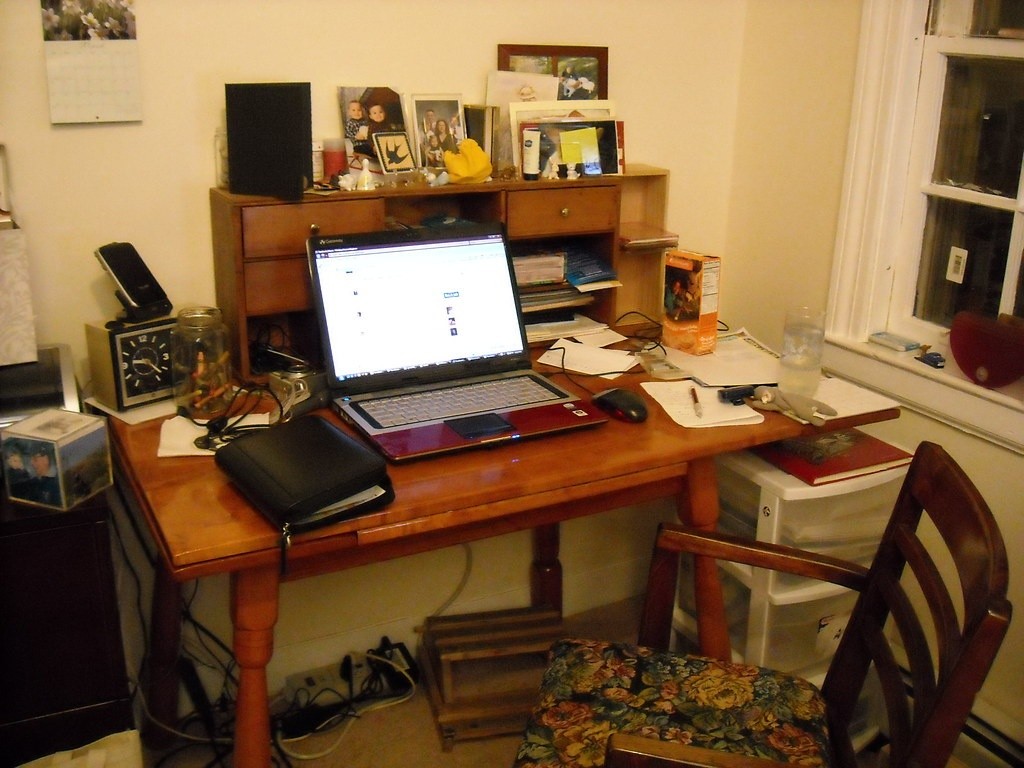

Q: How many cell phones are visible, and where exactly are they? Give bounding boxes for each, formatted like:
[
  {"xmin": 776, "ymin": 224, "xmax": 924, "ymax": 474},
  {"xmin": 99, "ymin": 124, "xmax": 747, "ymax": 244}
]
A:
[{"xmin": 96, "ymin": 241, "xmax": 168, "ymax": 308}]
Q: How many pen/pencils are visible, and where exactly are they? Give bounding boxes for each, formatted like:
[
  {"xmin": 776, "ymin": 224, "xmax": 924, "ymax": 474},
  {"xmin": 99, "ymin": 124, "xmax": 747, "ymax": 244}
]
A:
[
  {"xmin": 195, "ymin": 383, "xmax": 230, "ymax": 408},
  {"xmin": 175, "ymin": 351, "xmax": 230, "ymax": 401},
  {"xmin": 689, "ymin": 386, "xmax": 701, "ymax": 416}
]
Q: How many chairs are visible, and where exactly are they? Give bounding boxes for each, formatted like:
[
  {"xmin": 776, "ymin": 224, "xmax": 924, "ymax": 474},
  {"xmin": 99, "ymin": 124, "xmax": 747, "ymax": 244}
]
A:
[{"xmin": 513, "ymin": 437, "xmax": 1013, "ymax": 768}]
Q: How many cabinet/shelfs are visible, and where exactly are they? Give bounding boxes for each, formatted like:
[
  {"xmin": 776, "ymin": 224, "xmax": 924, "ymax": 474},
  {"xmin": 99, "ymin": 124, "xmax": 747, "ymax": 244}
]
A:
[
  {"xmin": 667, "ymin": 430, "xmax": 913, "ymax": 668},
  {"xmin": 210, "ymin": 163, "xmax": 680, "ymax": 390},
  {"xmin": 0, "ymin": 492, "xmax": 138, "ymax": 768}
]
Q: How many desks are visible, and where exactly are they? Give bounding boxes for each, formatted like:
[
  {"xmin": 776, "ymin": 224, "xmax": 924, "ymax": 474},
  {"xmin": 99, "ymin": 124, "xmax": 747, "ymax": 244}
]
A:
[{"xmin": 114, "ymin": 329, "xmax": 902, "ymax": 767}]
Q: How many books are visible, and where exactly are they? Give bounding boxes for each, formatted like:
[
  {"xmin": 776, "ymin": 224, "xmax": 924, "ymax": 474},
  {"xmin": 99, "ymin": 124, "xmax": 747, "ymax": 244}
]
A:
[
  {"xmin": 751, "ymin": 426, "xmax": 914, "ymax": 486},
  {"xmin": 510, "ymin": 245, "xmax": 622, "ymax": 345}
]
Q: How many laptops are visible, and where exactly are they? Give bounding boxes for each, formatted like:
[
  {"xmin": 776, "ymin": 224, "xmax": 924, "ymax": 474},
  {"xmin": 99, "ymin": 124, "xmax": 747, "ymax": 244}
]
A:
[{"xmin": 305, "ymin": 224, "xmax": 608, "ymax": 463}]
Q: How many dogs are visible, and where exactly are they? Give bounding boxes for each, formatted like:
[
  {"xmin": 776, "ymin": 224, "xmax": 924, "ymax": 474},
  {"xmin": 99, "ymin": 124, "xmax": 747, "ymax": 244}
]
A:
[{"xmin": 560, "ymin": 66, "xmax": 595, "ymax": 98}]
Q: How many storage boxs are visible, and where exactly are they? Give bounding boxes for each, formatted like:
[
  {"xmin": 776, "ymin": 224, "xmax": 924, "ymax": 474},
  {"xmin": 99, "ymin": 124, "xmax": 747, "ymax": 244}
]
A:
[
  {"xmin": 0, "ymin": 405, "xmax": 114, "ymax": 511},
  {"xmin": 663, "ymin": 247, "xmax": 721, "ymax": 354}
]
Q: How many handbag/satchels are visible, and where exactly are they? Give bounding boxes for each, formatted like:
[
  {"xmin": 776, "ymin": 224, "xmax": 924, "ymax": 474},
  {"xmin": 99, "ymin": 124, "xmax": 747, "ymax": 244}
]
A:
[{"xmin": 215, "ymin": 413, "xmax": 396, "ymax": 580}]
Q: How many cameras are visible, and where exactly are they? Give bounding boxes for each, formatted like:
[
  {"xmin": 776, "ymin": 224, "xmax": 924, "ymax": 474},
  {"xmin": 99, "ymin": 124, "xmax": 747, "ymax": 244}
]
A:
[{"xmin": 276, "ymin": 364, "xmax": 329, "ymax": 420}]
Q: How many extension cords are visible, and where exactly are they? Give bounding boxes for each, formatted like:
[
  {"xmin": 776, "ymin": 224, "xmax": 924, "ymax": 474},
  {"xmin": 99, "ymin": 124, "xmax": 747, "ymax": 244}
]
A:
[{"xmin": 286, "ymin": 641, "xmax": 422, "ymax": 710}]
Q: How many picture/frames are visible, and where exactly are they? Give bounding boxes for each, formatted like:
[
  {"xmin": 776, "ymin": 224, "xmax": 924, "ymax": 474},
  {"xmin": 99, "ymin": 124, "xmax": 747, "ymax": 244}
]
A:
[
  {"xmin": 370, "ymin": 133, "xmax": 417, "ymax": 175},
  {"xmin": 410, "ymin": 93, "xmax": 468, "ymax": 171},
  {"xmin": 463, "ymin": 106, "xmax": 493, "ymax": 178},
  {"xmin": 335, "ymin": 82, "xmax": 414, "ymax": 175},
  {"xmin": 497, "ymin": 44, "xmax": 608, "ymax": 103}
]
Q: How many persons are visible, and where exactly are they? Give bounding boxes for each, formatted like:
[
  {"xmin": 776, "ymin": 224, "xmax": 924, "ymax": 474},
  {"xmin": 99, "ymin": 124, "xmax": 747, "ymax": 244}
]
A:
[
  {"xmin": 663, "ymin": 279, "xmax": 693, "ymax": 323},
  {"xmin": 2, "ymin": 439, "xmax": 62, "ymax": 505},
  {"xmin": 345, "ymin": 99, "xmax": 398, "ymax": 160},
  {"xmin": 559, "ymin": 62, "xmax": 592, "ymax": 100},
  {"xmin": 421, "ymin": 107, "xmax": 459, "ymax": 168}
]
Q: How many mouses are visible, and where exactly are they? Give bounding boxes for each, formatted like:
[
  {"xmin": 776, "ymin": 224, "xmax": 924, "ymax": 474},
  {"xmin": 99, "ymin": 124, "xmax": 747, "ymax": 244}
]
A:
[{"xmin": 590, "ymin": 387, "xmax": 649, "ymax": 424}]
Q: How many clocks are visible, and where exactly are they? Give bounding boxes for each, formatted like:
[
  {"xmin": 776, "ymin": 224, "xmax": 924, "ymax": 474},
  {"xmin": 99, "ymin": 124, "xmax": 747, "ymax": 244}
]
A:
[{"xmin": 109, "ymin": 318, "xmax": 176, "ymax": 408}]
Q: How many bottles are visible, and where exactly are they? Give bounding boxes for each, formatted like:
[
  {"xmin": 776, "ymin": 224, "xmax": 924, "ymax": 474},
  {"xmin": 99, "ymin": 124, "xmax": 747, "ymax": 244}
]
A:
[
  {"xmin": 169, "ymin": 306, "xmax": 233, "ymax": 420},
  {"xmin": 357, "ymin": 159, "xmax": 376, "ymax": 191}
]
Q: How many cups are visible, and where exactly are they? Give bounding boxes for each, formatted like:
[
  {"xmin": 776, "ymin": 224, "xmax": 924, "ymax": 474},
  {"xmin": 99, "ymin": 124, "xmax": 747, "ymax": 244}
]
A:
[
  {"xmin": 523, "ymin": 128, "xmax": 541, "ymax": 180},
  {"xmin": 323, "ymin": 138, "xmax": 346, "ymax": 181},
  {"xmin": 779, "ymin": 306, "xmax": 828, "ymax": 399}
]
